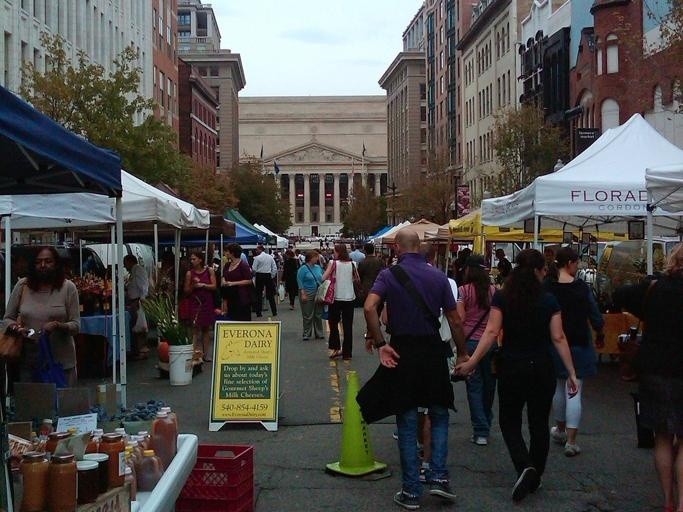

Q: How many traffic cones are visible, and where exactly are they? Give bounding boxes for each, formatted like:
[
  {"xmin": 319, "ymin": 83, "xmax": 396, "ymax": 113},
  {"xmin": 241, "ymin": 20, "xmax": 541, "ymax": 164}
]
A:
[{"xmin": 323, "ymin": 368, "xmax": 387, "ymax": 478}]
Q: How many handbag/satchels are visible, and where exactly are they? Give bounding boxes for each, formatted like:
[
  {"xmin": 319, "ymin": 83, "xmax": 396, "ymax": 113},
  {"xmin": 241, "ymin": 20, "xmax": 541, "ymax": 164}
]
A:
[
  {"xmin": 448, "ymin": 347, "xmax": 471, "ymax": 381},
  {"xmin": 1, "ymin": 279, "xmax": 26, "ymax": 363},
  {"xmin": 33, "ymin": 333, "xmax": 67, "ymax": 387},
  {"xmin": 206, "ymin": 267, "xmax": 221, "ymax": 307},
  {"xmin": 615, "ymin": 328, "xmax": 645, "ymax": 381},
  {"xmin": 314, "ymin": 259, "xmax": 337, "ymax": 305},
  {"xmin": 322, "ymin": 305, "xmax": 329, "ymax": 320},
  {"xmin": 350, "ymin": 260, "xmax": 361, "ymax": 297},
  {"xmin": 250, "ymin": 280, "xmax": 257, "ymax": 305}
]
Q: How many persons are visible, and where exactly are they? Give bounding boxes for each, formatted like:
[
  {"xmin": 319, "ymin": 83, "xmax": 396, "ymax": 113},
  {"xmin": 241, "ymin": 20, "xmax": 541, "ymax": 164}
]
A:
[
  {"xmin": 123, "ymin": 254, "xmax": 149, "ymax": 360},
  {"xmin": 2, "ymin": 245, "xmax": 81, "ymax": 417},
  {"xmin": 613, "ymin": 242, "xmax": 683, "ymax": 512},
  {"xmin": 164, "ymin": 226, "xmax": 607, "ymax": 510}
]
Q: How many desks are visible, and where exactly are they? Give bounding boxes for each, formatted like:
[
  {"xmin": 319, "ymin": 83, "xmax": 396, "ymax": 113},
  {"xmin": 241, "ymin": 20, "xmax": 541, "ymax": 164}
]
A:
[{"xmin": 75, "ymin": 310, "xmax": 131, "ymax": 377}]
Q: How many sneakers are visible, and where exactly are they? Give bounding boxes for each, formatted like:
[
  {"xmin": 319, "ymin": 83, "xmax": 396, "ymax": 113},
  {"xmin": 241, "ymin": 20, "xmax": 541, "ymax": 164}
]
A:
[
  {"xmin": 429, "ymin": 480, "xmax": 456, "ymax": 499},
  {"xmin": 418, "ymin": 465, "xmax": 432, "ymax": 483},
  {"xmin": 564, "ymin": 441, "xmax": 580, "ymax": 456},
  {"xmin": 393, "ymin": 488, "xmax": 420, "ymax": 510},
  {"xmin": 470, "ymin": 434, "xmax": 488, "ymax": 445},
  {"xmin": 511, "ymin": 464, "xmax": 536, "ymax": 501},
  {"xmin": 550, "ymin": 426, "xmax": 567, "ymax": 443}
]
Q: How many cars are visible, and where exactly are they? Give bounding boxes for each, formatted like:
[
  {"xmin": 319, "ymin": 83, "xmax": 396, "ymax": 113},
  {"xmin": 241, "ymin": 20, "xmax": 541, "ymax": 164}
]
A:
[{"xmin": 279, "ymin": 232, "xmax": 358, "ymax": 244}]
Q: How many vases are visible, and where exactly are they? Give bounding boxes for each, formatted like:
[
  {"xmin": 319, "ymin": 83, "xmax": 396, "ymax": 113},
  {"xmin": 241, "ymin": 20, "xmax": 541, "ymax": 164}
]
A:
[
  {"xmin": 83, "ymin": 293, "xmax": 95, "ymax": 315},
  {"xmin": 99, "ymin": 294, "xmax": 112, "ymax": 314}
]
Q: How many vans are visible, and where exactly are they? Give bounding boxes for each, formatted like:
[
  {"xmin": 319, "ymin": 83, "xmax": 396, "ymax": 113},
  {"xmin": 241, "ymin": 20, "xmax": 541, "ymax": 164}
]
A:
[
  {"xmin": 490, "ymin": 235, "xmax": 679, "ymax": 297},
  {"xmin": 10, "ymin": 242, "xmax": 104, "ymax": 278}
]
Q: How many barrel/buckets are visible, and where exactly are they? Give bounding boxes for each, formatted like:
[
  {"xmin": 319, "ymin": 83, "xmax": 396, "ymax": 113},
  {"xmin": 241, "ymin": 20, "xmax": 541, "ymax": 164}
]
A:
[{"xmin": 166, "ymin": 344, "xmax": 195, "ymax": 386}]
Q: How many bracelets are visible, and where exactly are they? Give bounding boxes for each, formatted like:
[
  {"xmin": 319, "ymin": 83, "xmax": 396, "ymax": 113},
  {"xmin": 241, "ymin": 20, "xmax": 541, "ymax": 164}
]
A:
[{"xmin": 12, "ymin": 325, "xmax": 16, "ymax": 332}]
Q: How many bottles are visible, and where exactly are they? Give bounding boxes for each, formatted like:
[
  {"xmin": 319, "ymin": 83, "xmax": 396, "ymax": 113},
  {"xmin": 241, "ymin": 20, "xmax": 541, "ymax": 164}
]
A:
[
  {"xmin": 13, "ymin": 406, "xmax": 178, "ymax": 502},
  {"xmin": 68, "ymin": 272, "xmax": 126, "ymax": 315}
]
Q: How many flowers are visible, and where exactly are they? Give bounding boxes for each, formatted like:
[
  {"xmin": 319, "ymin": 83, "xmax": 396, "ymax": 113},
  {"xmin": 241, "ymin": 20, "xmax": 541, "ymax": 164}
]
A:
[
  {"xmin": 99, "ymin": 279, "xmax": 112, "ymax": 296},
  {"xmin": 71, "ymin": 273, "xmax": 105, "ymax": 295}
]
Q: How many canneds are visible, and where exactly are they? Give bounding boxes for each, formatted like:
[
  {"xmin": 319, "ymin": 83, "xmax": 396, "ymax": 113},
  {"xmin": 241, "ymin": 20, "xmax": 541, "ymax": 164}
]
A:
[
  {"xmin": 76, "ymin": 461, "xmax": 99, "ymax": 503},
  {"xmin": 99, "ymin": 433, "xmax": 126, "ymax": 488},
  {"xmin": 85, "ymin": 434, "xmax": 97, "ymax": 453},
  {"xmin": 49, "ymin": 453, "xmax": 78, "ymax": 510},
  {"xmin": 19, "ymin": 451, "xmax": 50, "ymax": 512},
  {"xmin": 45, "ymin": 432, "xmax": 72, "ymax": 453},
  {"xmin": 83, "ymin": 452, "xmax": 109, "ymax": 493}
]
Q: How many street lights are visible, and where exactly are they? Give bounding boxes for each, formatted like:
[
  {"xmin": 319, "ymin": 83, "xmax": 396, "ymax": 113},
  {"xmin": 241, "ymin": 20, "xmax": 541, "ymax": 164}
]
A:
[
  {"xmin": 386, "ymin": 182, "xmax": 398, "ymax": 228},
  {"xmin": 451, "ymin": 173, "xmax": 460, "ymax": 218},
  {"xmin": 554, "ymin": 158, "xmax": 564, "ymax": 173}
]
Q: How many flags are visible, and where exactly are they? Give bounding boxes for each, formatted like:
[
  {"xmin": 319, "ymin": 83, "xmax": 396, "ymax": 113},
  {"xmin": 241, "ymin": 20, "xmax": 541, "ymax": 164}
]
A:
[
  {"xmin": 274, "ymin": 162, "xmax": 279, "ymax": 173},
  {"xmin": 260, "ymin": 144, "xmax": 263, "ymax": 158},
  {"xmin": 362, "ymin": 142, "xmax": 366, "ymax": 156},
  {"xmin": 348, "ymin": 164, "xmax": 354, "ymax": 177}
]
